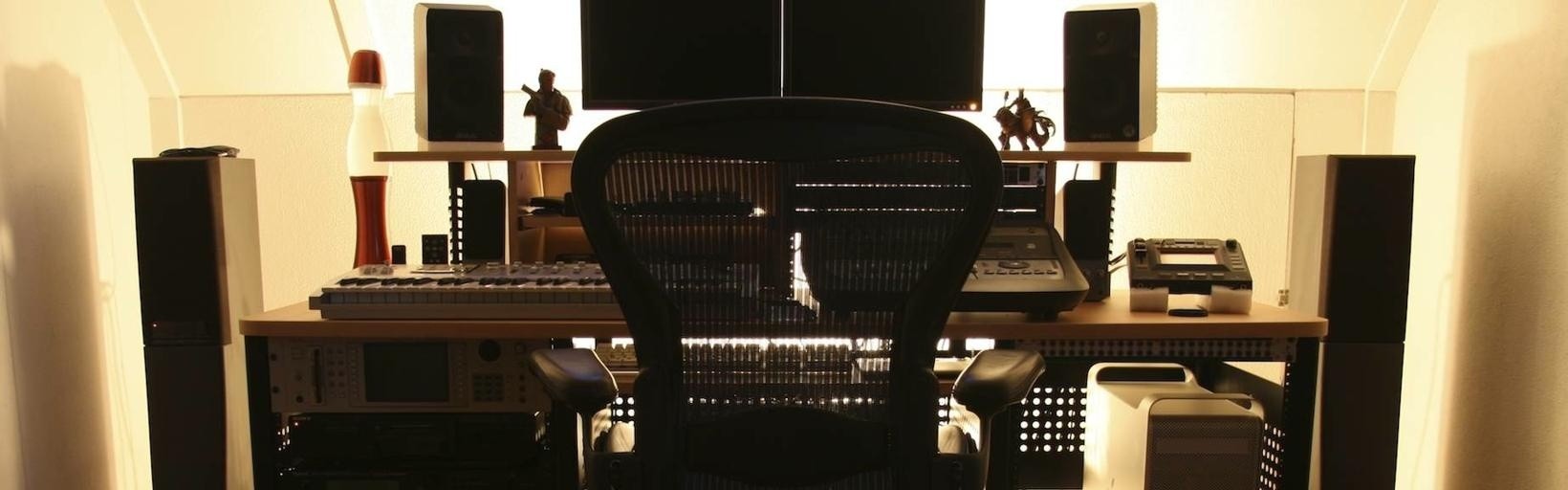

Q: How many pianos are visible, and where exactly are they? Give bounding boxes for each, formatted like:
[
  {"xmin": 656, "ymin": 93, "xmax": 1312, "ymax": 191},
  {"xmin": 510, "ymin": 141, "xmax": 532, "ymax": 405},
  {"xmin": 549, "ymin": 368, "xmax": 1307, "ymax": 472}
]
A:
[{"xmin": 308, "ymin": 262, "xmax": 623, "ymax": 319}]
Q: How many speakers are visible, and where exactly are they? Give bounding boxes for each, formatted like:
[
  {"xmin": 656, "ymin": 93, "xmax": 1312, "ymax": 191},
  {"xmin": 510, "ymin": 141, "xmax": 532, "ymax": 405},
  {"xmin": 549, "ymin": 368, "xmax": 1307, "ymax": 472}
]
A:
[
  {"xmin": 1286, "ymin": 151, "xmax": 1420, "ymax": 345},
  {"xmin": 1060, "ymin": 0, "xmax": 1161, "ymax": 143},
  {"xmin": 412, "ymin": 0, "xmax": 507, "ymax": 157},
  {"xmin": 1309, "ymin": 329, "xmax": 1408, "ymax": 490},
  {"xmin": 131, "ymin": 157, "xmax": 264, "ymax": 350},
  {"xmin": 141, "ymin": 345, "xmax": 256, "ymax": 490},
  {"xmin": 462, "ymin": 179, "xmax": 506, "ymax": 263},
  {"xmin": 1052, "ymin": 180, "xmax": 1111, "ymax": 302}
]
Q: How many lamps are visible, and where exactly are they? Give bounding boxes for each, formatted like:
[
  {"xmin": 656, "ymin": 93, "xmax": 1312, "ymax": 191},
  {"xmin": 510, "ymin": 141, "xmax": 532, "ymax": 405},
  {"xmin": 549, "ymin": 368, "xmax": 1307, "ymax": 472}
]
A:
[{"xmin": 349, "ymin": 49, "xmax": 392, "ymax": 270}]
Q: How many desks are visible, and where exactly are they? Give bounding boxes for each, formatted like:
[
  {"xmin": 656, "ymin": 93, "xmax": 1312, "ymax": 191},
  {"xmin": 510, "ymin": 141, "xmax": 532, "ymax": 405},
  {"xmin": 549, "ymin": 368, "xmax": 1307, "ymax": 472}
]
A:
[{"xmin": 240, "ymin": 152, "xmax": 1329, "ymax": 489}]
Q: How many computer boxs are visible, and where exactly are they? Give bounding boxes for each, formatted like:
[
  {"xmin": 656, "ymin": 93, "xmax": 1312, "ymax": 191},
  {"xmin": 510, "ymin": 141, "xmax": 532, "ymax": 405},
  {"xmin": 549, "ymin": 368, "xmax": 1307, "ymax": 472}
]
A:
[{"xmin": 1082, "ymin": 363, "xmax": 1268, "ymax": 488}]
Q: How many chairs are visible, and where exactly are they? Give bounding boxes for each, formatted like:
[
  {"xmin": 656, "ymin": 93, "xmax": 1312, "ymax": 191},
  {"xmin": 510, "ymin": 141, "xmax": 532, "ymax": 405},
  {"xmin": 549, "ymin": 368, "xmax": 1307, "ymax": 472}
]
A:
[{"xmin": 527, "ymin": 95, "xmax": 1046, "ymax": 490}]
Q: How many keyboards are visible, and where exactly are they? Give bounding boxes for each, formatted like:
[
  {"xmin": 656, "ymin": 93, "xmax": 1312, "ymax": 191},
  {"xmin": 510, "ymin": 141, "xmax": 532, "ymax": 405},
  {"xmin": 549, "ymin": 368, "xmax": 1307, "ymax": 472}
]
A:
[{"xmin": 592, "ymin": 338, "xmax": 851, "ymax": 372}]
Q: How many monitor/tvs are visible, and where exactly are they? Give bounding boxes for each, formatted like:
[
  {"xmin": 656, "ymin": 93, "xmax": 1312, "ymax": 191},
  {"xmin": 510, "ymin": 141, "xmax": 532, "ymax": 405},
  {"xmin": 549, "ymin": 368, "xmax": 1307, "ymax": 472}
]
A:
[
  {"xmin": 580, "ymin": 0, "xmax": 778, "ymax": 109},
  {"xmin": 782, "ymin": 1, "xmax": 988, "ymax": 116}
]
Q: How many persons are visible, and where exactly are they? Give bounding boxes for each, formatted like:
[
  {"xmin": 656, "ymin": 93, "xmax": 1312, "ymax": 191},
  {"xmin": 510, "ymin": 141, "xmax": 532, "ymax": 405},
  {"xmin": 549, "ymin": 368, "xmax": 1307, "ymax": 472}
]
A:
[
  {"xmin": 1007, "ymin": 88, "xmax": 1031, "ymax": 115},
  {"xmin": 521, "ymin": 69, "xmax": 571, "ymax": 146}
]
